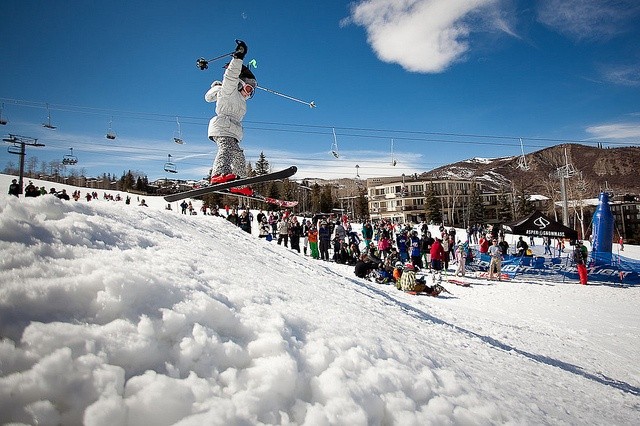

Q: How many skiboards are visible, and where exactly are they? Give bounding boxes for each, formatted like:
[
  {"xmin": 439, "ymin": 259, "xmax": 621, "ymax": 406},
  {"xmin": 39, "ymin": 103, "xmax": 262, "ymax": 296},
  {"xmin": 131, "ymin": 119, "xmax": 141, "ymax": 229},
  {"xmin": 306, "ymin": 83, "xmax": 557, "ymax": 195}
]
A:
[{"xmin": 163, "ymin": 166, "xmax": 298, "ymax": 209}]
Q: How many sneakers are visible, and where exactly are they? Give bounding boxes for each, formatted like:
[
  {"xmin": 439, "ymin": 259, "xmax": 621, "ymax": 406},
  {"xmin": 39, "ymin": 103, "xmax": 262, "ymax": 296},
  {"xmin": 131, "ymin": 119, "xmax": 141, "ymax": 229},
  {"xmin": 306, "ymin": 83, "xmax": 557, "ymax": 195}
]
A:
[
  {"xmin": 230, "ymin": 187, "xmax": 254, "ymax": 196},
  {"xmin": 211, "ymin": 174, "xmax": 237, "ymax": 184}
]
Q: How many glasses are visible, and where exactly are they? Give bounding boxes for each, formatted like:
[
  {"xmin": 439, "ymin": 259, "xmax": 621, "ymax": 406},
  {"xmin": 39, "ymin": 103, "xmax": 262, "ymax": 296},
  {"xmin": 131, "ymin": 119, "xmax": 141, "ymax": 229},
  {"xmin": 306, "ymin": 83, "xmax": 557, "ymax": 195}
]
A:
[{"xmin": 239, "ymin": 78, "xmax": 254, "ymax": 95}]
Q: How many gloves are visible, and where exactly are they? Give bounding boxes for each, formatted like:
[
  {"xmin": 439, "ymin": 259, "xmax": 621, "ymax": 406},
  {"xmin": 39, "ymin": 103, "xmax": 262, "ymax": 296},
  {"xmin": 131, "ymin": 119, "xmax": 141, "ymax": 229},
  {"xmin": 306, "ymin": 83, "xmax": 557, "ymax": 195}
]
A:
[{"xmin": 231, "ymin": 40, "xmax": 247, "ymax": 60}]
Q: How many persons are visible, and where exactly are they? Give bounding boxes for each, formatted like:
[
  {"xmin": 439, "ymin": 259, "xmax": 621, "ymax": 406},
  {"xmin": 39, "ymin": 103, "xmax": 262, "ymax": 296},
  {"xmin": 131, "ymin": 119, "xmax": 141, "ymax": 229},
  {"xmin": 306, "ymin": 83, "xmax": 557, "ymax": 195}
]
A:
[
  {"xmin": 62, "ymin": 156, "xmax": 77, "ymax": 164},
  {"xmin": 7, "ymin": 177, "xmax": 148, "ymax": 209},
  {"xmin": 166, "ymin": 200, "xmax": 359, "ymax": 266},
  {"xmin": 357, "ymin": 210, "xmax": 590, "ymax": 296},
  {"xmin": 205, "ymin": 40, "xmax": 257, "ymax": 197},
  {"xmin": 618, "ymin": 235, "xmax": 624, "ymax": 251}
]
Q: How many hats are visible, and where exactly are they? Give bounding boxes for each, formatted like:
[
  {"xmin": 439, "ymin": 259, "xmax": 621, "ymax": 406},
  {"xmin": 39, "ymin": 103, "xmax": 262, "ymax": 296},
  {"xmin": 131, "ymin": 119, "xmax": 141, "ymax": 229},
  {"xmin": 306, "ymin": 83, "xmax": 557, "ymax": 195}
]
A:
[{"xmin": 222, "ymin": 62, "xmax": 257, "ymax": 91}]
